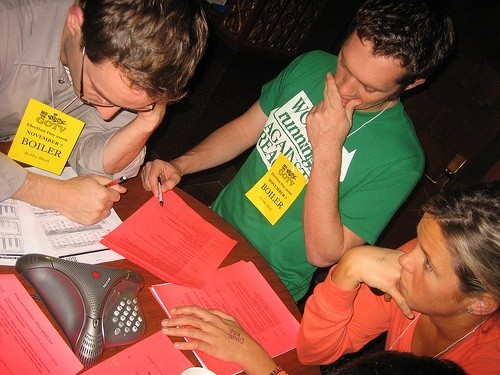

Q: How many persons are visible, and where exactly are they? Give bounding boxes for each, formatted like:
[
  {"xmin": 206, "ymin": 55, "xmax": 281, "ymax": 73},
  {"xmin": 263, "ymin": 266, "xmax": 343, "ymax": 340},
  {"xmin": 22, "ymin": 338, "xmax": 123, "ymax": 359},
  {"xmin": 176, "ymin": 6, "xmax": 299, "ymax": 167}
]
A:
[
  {"xmin": 139, "ymin": 0, "xmax": 454, "ymax": 304},
  {"xmin": 159, "ymin": 177, "xmax": 500, "ymax": 375},
  {"xmin": 1, "ymin": 0, "xmax": 207, "ymax": 226}
]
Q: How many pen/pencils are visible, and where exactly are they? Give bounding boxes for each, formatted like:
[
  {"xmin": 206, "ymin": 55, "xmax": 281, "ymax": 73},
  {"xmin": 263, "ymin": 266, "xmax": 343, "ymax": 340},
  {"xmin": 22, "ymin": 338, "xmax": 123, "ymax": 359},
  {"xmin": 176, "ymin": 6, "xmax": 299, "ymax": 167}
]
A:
[
  {"xmin": 105, "ymin": 176, "xmax": 126, "ymax": 187},
  {"xmin": 157, "ymin": 175, "xmax": 164, "ymax": 207}
]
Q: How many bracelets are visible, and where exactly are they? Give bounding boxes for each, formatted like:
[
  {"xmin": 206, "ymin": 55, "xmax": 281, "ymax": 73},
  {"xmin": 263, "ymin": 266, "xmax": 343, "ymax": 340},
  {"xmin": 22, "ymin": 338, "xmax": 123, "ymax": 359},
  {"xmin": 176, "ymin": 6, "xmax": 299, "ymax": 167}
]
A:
[{"xmin": 268, "ymin": 365, "xmax": 283, "ymax": 375}]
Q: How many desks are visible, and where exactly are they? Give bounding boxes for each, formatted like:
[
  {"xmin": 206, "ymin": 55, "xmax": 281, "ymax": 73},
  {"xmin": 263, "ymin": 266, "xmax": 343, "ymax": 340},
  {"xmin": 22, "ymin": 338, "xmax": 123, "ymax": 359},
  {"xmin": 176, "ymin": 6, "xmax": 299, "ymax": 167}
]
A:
[{"xmin": 0, "ymin": 139, "xmax": 322, "ymax": 375}]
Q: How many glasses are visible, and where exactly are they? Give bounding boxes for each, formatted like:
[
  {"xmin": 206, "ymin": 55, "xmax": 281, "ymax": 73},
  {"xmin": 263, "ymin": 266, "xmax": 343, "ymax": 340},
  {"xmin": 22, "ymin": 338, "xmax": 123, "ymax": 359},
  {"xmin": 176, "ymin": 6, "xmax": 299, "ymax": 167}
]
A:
[{"xmin": 79, "ymin": 45, "xmax": 157, "ymax": 112}]
